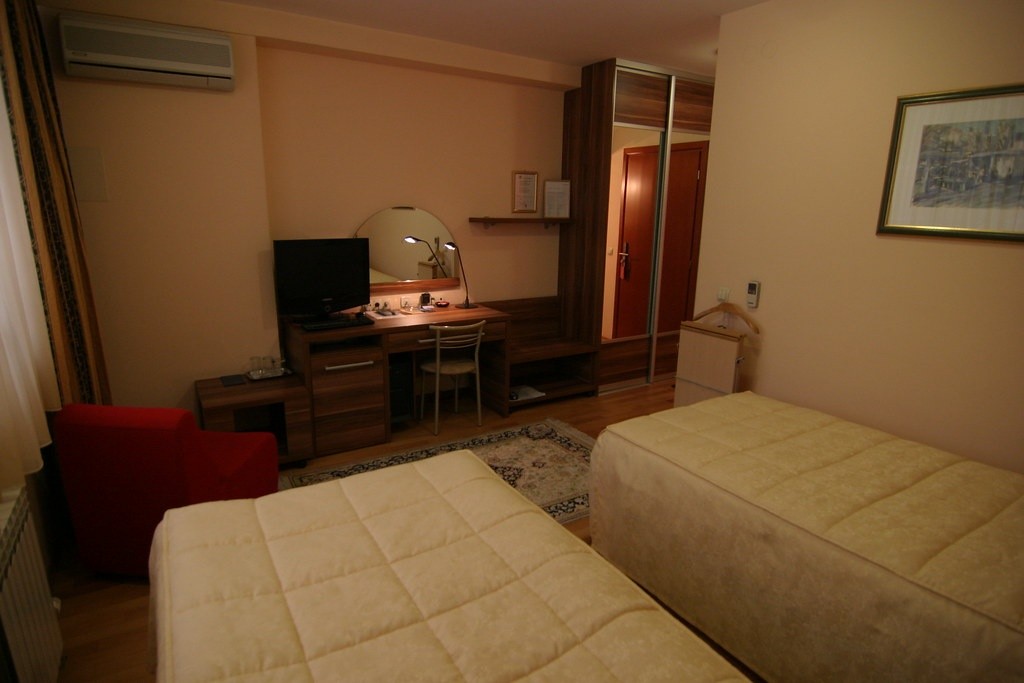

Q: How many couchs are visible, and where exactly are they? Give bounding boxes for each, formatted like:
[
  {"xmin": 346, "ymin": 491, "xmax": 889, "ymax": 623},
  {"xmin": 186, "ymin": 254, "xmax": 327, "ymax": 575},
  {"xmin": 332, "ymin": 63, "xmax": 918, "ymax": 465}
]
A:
[{"xmin": 52, "ymin": 402, "xmax": 280, "ymax": 575}]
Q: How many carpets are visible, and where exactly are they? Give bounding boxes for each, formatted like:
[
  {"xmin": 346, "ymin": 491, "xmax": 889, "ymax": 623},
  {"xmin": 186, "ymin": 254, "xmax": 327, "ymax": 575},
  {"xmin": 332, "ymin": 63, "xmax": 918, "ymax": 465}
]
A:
[{"xmin": 293, "ymin": 418, "xmax": 597, "ymax": 527}]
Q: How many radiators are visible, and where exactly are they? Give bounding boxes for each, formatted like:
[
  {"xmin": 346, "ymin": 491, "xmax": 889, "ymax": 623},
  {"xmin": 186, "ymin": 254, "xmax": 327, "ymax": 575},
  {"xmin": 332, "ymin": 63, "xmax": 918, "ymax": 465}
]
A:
[{"xmin": 0, "ymin": 483, "xmax": 64, "ymax": 683}]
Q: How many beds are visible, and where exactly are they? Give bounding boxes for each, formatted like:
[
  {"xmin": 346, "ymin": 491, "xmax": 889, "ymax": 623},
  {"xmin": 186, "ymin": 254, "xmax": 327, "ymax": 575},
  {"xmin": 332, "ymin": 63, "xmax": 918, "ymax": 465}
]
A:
[
  {"xmin": 582, "ymin": 389, "xmax": 1024, "ymax": 683},
  {"xmin": 138, "ymin": 447, "xmax": 752, "ymax": 683}
]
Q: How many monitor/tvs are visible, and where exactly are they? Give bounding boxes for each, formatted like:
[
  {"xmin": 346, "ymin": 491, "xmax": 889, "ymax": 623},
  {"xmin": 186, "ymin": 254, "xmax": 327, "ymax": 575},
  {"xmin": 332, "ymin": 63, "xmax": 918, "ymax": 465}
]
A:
[{"xmin": 273, "ymin": 238, "xmax": 370, "ymax": 325}]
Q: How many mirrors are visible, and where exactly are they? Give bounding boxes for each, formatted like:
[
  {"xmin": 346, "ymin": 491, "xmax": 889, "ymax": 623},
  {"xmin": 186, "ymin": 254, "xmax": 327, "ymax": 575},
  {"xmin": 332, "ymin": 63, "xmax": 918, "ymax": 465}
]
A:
[
  {"xmin": 352, "ymin": 205, "xmax": 459, "ymax": 282},
  {"xmin": 600, "ymin": 121, "xmax": 711, "ymax": 341}
]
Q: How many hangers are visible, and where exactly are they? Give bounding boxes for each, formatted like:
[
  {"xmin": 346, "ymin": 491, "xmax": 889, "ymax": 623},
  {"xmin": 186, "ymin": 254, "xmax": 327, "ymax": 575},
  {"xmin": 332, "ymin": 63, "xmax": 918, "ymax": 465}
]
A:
[{"xmin": 691, "ymin": 287, "xmax": 762, "ymax": 336}]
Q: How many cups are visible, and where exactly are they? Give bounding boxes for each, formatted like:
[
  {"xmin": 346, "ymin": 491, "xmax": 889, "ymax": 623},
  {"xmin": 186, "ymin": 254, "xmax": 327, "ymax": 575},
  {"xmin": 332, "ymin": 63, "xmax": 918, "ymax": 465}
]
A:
[
  {"xmin": 400, "ymin": 296, "xmax": 413, "ymax": 312},
  {"xmin": 263, "ymin": 356, "xmax": 275, "ymax": 378},
  {"xmin": 250, "ymin": 356, "xmax": 263, "ymax": 378}
]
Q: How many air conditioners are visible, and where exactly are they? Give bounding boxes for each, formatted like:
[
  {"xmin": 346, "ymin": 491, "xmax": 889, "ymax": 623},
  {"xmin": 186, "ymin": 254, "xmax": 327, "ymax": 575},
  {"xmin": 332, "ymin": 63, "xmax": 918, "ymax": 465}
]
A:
[{"xmin": 51, "ymin": 7, "xmax": 236, "ymax": 90}]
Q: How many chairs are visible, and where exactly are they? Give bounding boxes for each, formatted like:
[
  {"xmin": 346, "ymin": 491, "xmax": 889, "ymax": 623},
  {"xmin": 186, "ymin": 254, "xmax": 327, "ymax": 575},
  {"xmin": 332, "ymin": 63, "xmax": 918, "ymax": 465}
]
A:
[{"xmin": 418, "ymin": 320, "xmax": 487, "ymax": 436}]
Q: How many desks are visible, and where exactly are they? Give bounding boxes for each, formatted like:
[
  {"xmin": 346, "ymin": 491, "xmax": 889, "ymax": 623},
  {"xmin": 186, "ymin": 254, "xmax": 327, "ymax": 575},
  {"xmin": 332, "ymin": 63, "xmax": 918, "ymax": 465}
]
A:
[
  {"xmin": 281, "ymin": 303, "xmax": 511, "ymax": 458},
  {"xmin": 193, "ymin": 371, "xmax": 315, "ymax": 465}
]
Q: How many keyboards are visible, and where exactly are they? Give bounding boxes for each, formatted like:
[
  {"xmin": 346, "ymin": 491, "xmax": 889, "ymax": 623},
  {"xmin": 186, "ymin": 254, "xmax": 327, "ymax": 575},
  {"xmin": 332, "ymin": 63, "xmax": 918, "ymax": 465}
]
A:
[{"xmin": 299, "ymin": 314, "xmax": 374, "ymax": 332}]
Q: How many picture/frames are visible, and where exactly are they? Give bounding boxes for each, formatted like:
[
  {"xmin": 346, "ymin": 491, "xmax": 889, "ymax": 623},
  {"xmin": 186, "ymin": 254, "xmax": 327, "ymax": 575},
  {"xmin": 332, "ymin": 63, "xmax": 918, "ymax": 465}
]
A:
[
  {"xmin": 543, "ymin": 179, "xmax": 571, "ymax": 218},
  {"xmin": 874, "ymin": 83, "xmax": 1024, "ymax": 242},
  {"xmin": 511, "ymin": 171, "xmax": 539, "ymax": 213}
]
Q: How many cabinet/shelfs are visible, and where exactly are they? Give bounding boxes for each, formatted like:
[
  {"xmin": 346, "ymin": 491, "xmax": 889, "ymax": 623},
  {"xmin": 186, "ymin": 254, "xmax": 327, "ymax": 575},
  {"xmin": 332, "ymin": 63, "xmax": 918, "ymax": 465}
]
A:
[
  {"xmin": 554, "ymin": 55, "xmax": 716, "ymax": 387},
  {"xmin": 506, "ymin": 335, "xmax": 600, "ymax": 418}
]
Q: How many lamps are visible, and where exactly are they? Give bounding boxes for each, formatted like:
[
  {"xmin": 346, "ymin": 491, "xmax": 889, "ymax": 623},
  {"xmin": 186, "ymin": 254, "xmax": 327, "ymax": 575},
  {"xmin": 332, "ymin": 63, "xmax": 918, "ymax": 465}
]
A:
[
  {"xmin": 444, "ymin": 242, "xmax": 478, "ymax": 309},
  {"xmin": 404, "ymin": 236, "xmax": 448, "ymax": 279}
]
